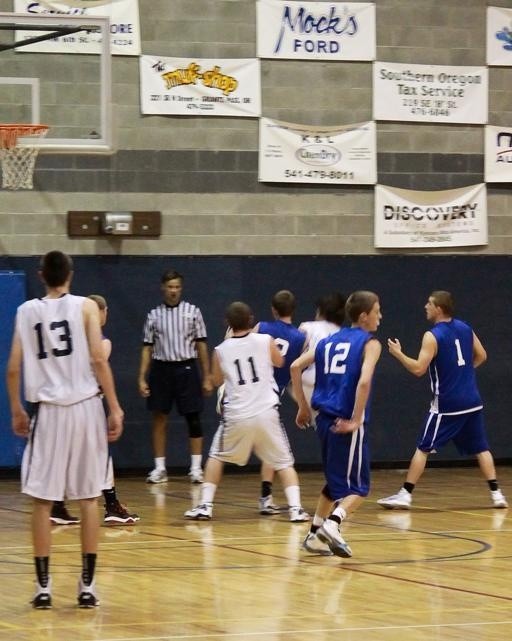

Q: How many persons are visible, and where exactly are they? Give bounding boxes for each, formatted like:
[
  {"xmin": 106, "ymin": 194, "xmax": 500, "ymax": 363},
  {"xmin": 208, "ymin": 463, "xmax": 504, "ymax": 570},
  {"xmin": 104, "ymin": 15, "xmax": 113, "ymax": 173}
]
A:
[
  {"xmin": 184, "ymin": 302, "xmax": 311, "ymax": 522},
  {"xmin": 287, "ymin": 292, "xmax": 346, "ymax": 508},
  {"xmin": 49, "ymin": 294, "xmax": 140, "ymax": 526},
  {"xmin": 290, "ymin": 290, "xmax": 383, "ymax": 557},
  {"xmin": 216, "ymin": 290, "xmax": 311, "ymax": 516},
  {"xmin": 138, "ymin": 271, "xmax": 214, "ymax": 484},
  {"xmin": 377, "ymin": 291, "xmax": 509, "ymax": 508},
  {"xmin": 6, "ymin": 251, "xmax": 124, "ymax": 609}
]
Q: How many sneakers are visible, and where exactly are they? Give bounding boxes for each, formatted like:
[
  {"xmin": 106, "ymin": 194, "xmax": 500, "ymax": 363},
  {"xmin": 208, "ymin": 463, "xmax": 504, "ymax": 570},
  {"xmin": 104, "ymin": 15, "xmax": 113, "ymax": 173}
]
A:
[
  {"xmin": 48, "ymin": 507, "xmax": 81, "ymax": 524},
  {"xmin": 259, "ymin": 494, "xmax": 310, "ymax": 522},
  {"xmin": 184, "ymin": 502, "xmax": 213, "ymax": 519},
  {"xmin": 29, "ymin": 575, "xmax": 52, "ymax": 609},
  {"xmin": 303, "ymin": 519, "xmax": 353, "ymax": 558},
  {"xmin": 104, "ymin": 500, "xmax": 141, "ymax": 522},
  {"xmin": 146, "ymin": 469, "xmax": 168, "ymax": 483},
  {"xmin": 376, "ymin": 487, "xmax": 413, "ymax": 509},
  {"xmin": 188, "ymin": 469, "xmax": 204, "ymax": 483},
  {"xmin": 490, "ymin": 488, "xmax": 509, "ymax": 507},
  {"xmin": 77, "ymin": 574, "xmax": 100, "ymax": 608}
]
